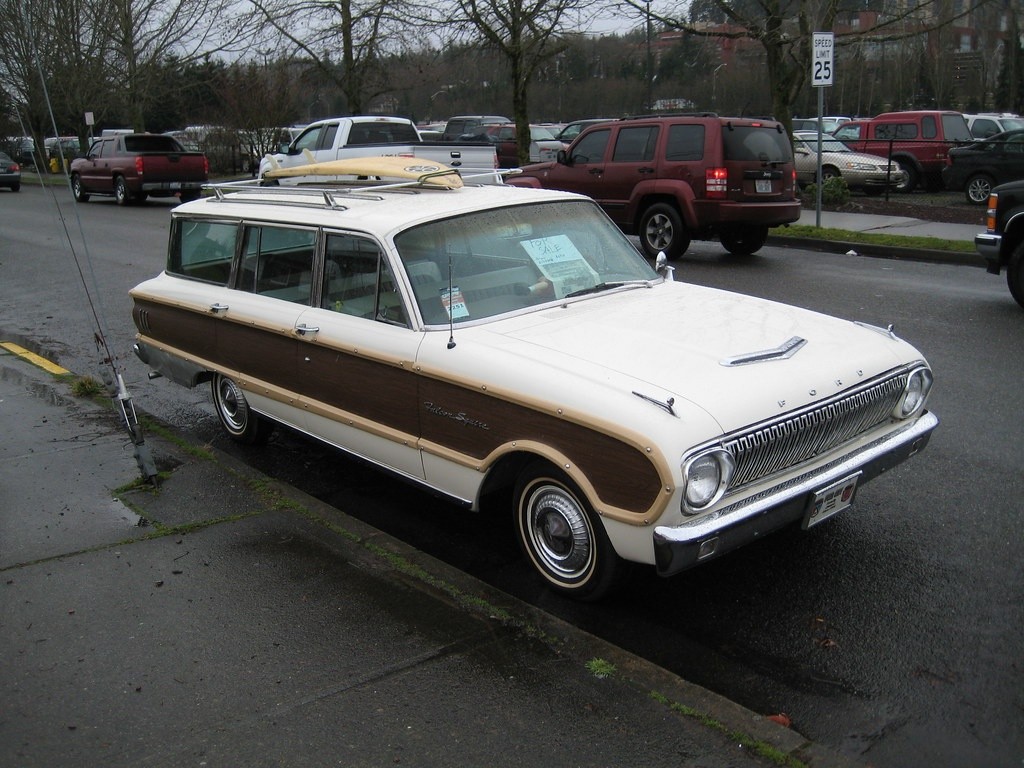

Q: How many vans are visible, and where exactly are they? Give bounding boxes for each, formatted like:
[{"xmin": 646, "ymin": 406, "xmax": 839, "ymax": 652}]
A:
[
  {"xmin": 830, "ymin": 110, "xmax": 975, "ymax": 194},
  {"xmin": 553, "ymin": 118, "xmax": 623, "ymax": 146},
  {"xmin": 102, "ymin": 130, "xmax": 150, "ymax": 138}
]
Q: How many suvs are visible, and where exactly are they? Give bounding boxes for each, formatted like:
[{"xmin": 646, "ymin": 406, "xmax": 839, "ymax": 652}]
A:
[
  {"xmin": 415, "ymin": 116, "xmax": 568, "ymax": 170},
  {"xmin": 502, "ymin": 111, "xmax": 803, "ymax": 262},
  {"xmin": 961, "ymin": 112, "xmax": 1024, "ymax": 144},
  {"xmin": 790, "ymin": 116, "xmax": 869, "ymax": 140},
  {"xmin": 164, "ymin": 126, "xmax": 307, "ymax": 172}
]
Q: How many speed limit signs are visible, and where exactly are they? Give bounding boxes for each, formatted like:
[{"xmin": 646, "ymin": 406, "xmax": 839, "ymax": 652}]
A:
[{"xmin": 811, "ymin": 31, "xmax": 835, "ymax": 88}]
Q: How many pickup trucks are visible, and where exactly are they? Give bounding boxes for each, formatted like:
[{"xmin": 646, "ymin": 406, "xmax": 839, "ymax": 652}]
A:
[
  {"xmin": 257, "ymin": 117, "xmax": 503, "ymax": 190},
  {"xmin": 69, "ymin": 134, "xmax": 208, "ymax": 208}
]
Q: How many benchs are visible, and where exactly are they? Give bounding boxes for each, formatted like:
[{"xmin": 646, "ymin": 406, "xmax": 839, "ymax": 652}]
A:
[{"xmin": 262, "ymin": 261, "xmax": 535, "ymax": 323}]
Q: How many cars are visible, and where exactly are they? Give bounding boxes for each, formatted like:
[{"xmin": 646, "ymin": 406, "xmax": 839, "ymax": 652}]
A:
[
  {"xmin": 941, "ymin": 128, "xmax": 1024, "ymax": 207},
  {"xmin": 0, "ymin": 152, "xmax": 22, "ymax": 192},
  {"xmin": 790, "ymin": 130, "xmax": 902, "ymax": 198},
  {"xmin": 973, "ymin": 180, "xmax": 1024, "ymax": 308},
  {"xmin": 4, "ymin": 135, "xmax": 101, "ymax": 160},
  {"xmin": 124, "ymin": 159, "xmax": 941, "ymax": 599}
]
